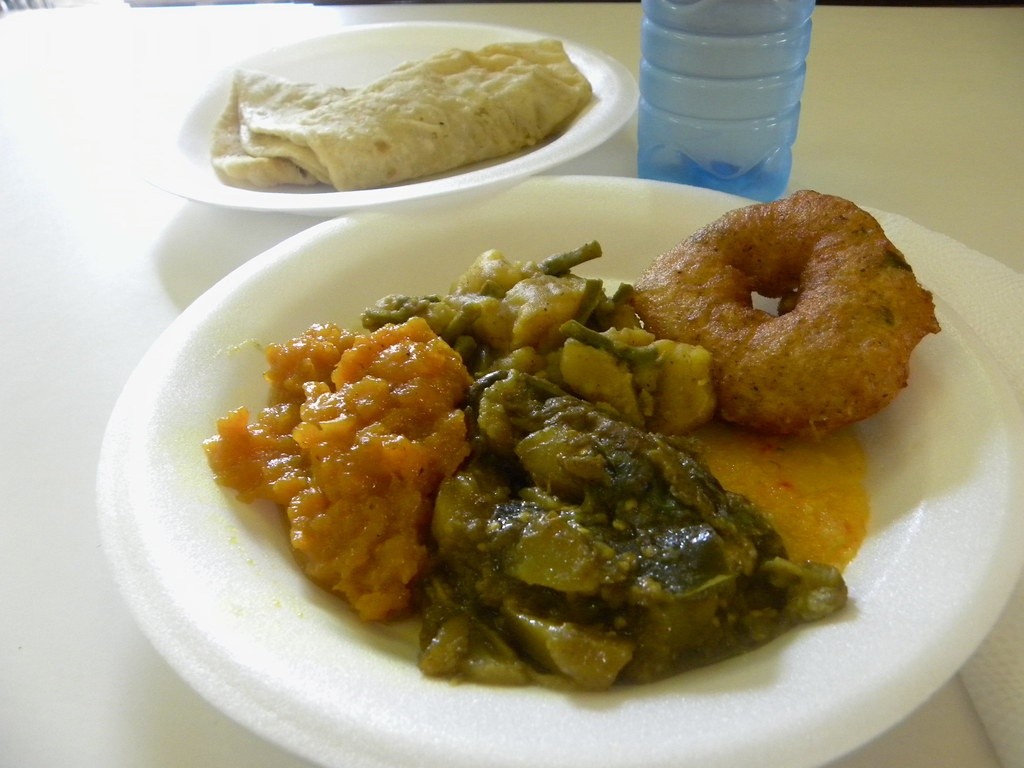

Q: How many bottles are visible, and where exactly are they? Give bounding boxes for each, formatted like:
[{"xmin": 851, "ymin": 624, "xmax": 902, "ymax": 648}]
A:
[{"xmin": 636, "ymin": 1, "xmax": 815, "ymax": 201}]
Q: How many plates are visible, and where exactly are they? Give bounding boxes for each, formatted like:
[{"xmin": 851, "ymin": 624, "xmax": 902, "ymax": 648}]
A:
[
  {"xmin": 171, "ymin": 21, "xmax": 640, "ymax": 215},
  {"xmin": 95, "ymin": 174, "xmax": 1024, "ymax": 768}
]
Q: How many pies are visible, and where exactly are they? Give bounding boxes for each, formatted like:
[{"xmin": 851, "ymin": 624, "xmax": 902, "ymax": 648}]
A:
[{"xmin": 208, "ymin": 37, "xmax": 594, "ymax": 192}]
